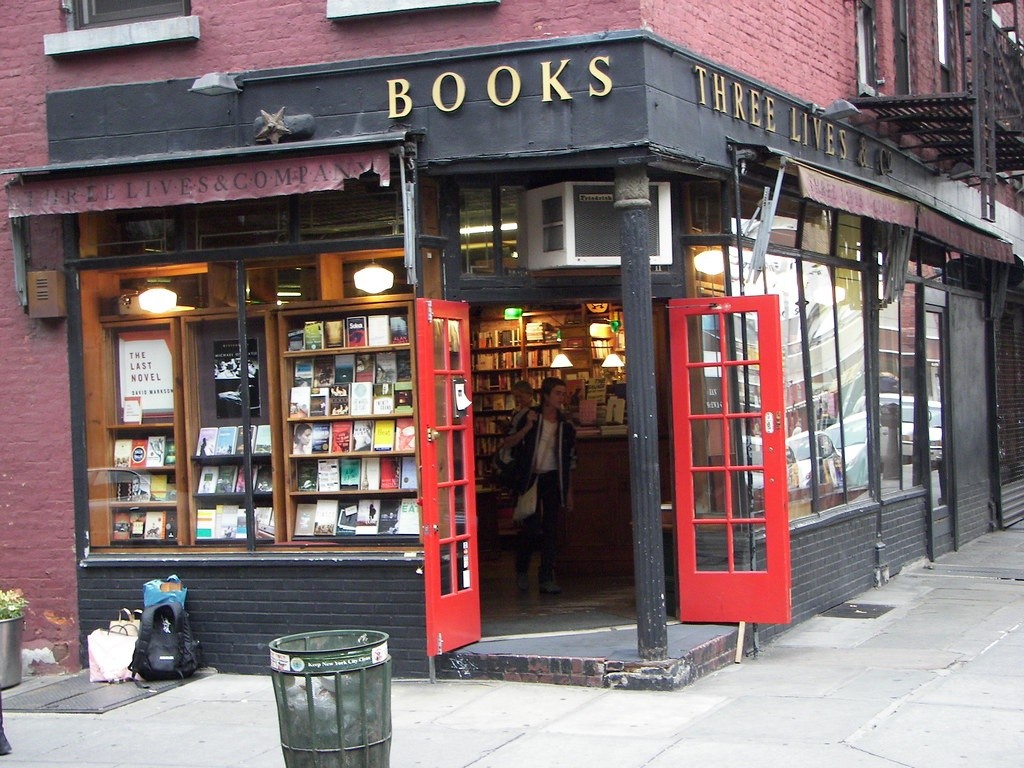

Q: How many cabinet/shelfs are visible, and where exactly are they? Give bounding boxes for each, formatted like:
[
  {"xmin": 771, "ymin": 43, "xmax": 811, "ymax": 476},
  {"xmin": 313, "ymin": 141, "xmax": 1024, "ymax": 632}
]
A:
[
  {"xmin": 99, "ymin": 319, "xmax": 187, "ymax": 549},
  {"xmin": 178, "ymin": 307, "xmax": 282, "ymax": 547},
  {"xmin": 522, "ymin": 306, "xmax": 591, "ymax": 405},
  {"xmin": 278, "ymin": 302, "xmax": 421, "ymax": 544},
  {"xmin": 471, "ymin": 309, "xmax": 522, "ymax": 553}
]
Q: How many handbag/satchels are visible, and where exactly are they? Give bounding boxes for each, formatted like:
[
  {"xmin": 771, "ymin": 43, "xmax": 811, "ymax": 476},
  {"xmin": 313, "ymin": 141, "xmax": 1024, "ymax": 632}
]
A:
[
  {"xmin": 88, "ymin": 607, "xmax": 144, "ymax": 683},
  {"xmin": 512, "ymin": 485, "xmax": 537, "ymax": 520},
  {"xmin": 143, "ymin": 574, "xmax": 187, "ymax": 608}
]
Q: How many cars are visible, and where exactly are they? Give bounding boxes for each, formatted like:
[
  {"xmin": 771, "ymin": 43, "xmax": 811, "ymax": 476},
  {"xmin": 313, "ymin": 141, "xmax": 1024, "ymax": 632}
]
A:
[
  {"xmin": 744, "ymin": 438, "xmax": 764, "ymax": 488},
  {"xmin": 786, "ymin": 430, "xmax": 843, "ymax": 487},
  {"xmin": 824, "ymin": 412, "xmax": 868, "ymax": 488},
  {"xmin": 876, "ymin": 395, "xmax": 943, "ymax": 464}
]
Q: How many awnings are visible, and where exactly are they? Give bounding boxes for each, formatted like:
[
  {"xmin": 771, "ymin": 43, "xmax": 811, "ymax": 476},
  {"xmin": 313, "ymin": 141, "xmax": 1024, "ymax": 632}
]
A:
[
  {"xmin": 0, "ymin": 127, "xmax": 429, "ymax": 219},
  {"xmin": 726, "ymin": 134, "xmax": 1016, "ymax": 264}
]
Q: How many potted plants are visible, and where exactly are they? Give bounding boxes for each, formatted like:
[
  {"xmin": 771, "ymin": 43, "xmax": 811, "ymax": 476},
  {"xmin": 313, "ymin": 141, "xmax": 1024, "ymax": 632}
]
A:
[{"xmin": 0, "ymin": 584, "xmax": 29, "ymax": 690}]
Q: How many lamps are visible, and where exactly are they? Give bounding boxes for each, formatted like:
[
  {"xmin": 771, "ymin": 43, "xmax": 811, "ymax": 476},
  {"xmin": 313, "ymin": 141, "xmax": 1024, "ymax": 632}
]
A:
[
  {"xmin": 504, "ymin": 307, "xmax": 523, "ymax": 320},
  {"xmin": 185, "ymin": 72, "xmax": 239, "ymax": 98},
  {"xmin": 600, "ymin": 348, "xmax": 625, "ymax": 369},
  {"xmin": 810, "ymin": 96, "xmax": 862, "ymax": 123},
  {"xmin": 134, "ymin": 280, "xmax": 179, "ymax": 313},
  {"xmin": 351, "ymin": 257, "xmax": 396, "ymax": 292},
  {"xmin": 549, "ymin": 345, "xmax": 574, "ymax": 369}
]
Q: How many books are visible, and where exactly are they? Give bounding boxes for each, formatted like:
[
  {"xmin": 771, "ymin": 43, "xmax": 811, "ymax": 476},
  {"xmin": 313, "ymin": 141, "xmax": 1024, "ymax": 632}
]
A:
[
  {"xmin": 195, "ymin": 425, "xmax": 278, "ymax": 540},
  {"xmin": 113, "ymin": 435, "xmax": 179, "ymax": 541},
  {"xmin": 288, "ymin": 314, "xmax": 422, "ymax": 537},
  {"xmin": 472, "ymin": 311, "xmax": 626, "ymax": 498}
]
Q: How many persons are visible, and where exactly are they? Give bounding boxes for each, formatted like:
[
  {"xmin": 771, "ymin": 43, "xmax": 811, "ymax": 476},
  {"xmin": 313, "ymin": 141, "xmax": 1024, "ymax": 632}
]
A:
[
  {"xmin": 292, "ymin": 423, "xmax": 312, "ymax": 455},
  {"xmin": 502, "ymin": 377, "xmax": 577, "ymax": 592},
  {"xmin": 792, "ymin": 422, "xmax": 802, "ymax": 436},
  {"xmin": 508, "ymin": 381, "xmax": 541, "ymax": 436}
]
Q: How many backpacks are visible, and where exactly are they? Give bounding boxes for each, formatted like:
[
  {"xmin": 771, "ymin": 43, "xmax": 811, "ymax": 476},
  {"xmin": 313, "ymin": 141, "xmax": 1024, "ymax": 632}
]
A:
[
  {"xmin": 492, "ymin": 407, "xmax": 537, "ymax": 487},
  {"xmin": 128, "ymin": 601, "xmax": 199, "ymax": 680}
]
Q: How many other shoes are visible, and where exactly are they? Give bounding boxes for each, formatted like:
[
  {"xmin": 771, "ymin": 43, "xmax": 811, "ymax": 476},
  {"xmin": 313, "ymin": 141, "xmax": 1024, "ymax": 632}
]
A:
[
  {"xmin": 516, "ymin": 572, "xmax": 530, "ymax": 591},
  {"xmin": 539, "ymin": 580, "xmax": 562, "ymax": 593}
]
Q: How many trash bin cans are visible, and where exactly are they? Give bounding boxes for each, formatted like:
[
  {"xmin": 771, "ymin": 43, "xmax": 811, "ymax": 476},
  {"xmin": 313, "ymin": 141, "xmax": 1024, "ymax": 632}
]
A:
[{"xmin": 267, "ymin": 630, "xmax": 393, "ymax": 768}]
